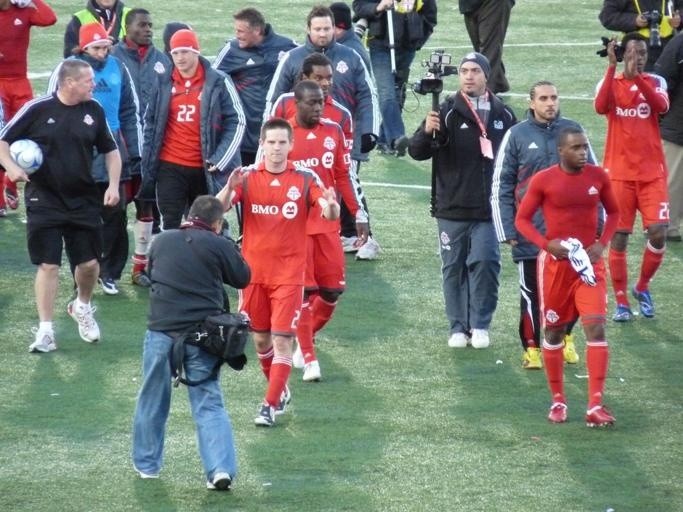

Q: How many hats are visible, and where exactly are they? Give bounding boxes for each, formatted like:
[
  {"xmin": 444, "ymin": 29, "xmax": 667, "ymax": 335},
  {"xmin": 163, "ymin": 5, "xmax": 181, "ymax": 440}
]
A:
[
  {"xmin": 80, "ymin": 24, "xmax": 112, "ymax": 50},
  {"xmin": 163, "ymin": 22, "xmax": 190, "ymax": 44},
  {"xmin": 460, "ymin": 54, "xmax": 489, "ymax": 79},
  {"xmin": 170, "ymin": 31, "xmax": 200, "ymax": 54},
  {"xmin": 330, "ymin": 2, "xmax": 351, "ymax": 29}
]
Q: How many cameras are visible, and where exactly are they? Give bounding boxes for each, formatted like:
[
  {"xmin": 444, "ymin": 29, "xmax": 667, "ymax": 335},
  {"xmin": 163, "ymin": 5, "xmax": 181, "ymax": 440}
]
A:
[
  {"xmin": 642, "ymin": 10, "xmax": 662, "ymax": 48},
  {"xmin": 413, "ymin": 49, "xmax": 458, "ymax": 95},
  {"xmin": 220, "ymin": 218, "xmax": 230, "ymax": 238},
  {"xmin": 354, "ymin": 17, "xmax": 368, "ymax": 41}
]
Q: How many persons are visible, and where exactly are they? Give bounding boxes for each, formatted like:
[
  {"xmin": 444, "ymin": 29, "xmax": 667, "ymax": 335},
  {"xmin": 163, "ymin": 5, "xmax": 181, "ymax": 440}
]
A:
[
  {"xmin": 490, "ymin": 81, "xmax": 605, "ymax": 370},
  {"xmin": 212, "ymin": 8, "xmax": 298, "ymax": 166},
  {"xmin": 595, "ymin": 36, "xmax": 671, "ymax": 320},
  {"xmin": 0, "ymin": 58, "xmax": 123, "ymax": 353},
  {"xmin": 132, "ymin": 30, "xmax": 247, "ymax": 232},
  {"xmin": 131, "ymin": 195, "xmax": 252, "ymax": 491},
  {"xmin": 264, "ymin": 5, "xmax": 384, "ymax": 261},
  {"xmin": 0, "ymin": 0, "xmax": 58, "ymax": 216},
  {"xmin": 64, "ymin": 1, "xmax": 131, "ymax": 59},
  {"xmin": 271, "ymin": 53, "xmax": 353, "ymax": 260},
  {"xmin": 459, "ymin": 0, "xmax": 515, "ymax": 93},
  {"xmin": 109, "ymin": 8, "xmax": 173, "ymax": 285},
  {"xmin": 514, "ymin": 129, "xmax": 621, "ymax": 426},
  {"xmin": 165, "ymin": 24, "xmax": 190, "ymax": 54},
  {"xmin": 330, "ymin": 1, "xmax": 371, "ymax": 77},
  {"xmin": 47, "ymin": 24, "xmax": 143, "ymax": 295},
  {"xmin": 598, "ymin": 1, "xmax": 683, "ymax": 72},
  {"xmin": 216, "ymin": 119, "xmax": 340, "ymax": 426},
  {"xmin": 409, "ymin": 52, "xmax": 517, "ymax": 348},
  {"xmin": 352, "ymin": 0, "xmax": 437, "ymax": 156},
  {"xmin": 262, "ymin": 81, "xmax": 369, "ymax": 380},
  {"xmin": 649, "ymin": 31, "xmax": 681, "ymax": 242}
]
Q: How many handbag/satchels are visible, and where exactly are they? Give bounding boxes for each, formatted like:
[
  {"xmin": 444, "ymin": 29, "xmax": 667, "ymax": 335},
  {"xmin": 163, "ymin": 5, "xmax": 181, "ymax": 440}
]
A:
[{"xmin": 201, "ymin": 314, "xmax": 249, "ymax": 360}]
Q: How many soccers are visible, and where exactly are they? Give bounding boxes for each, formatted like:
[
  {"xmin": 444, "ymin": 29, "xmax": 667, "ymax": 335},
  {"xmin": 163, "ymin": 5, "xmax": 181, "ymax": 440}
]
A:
[{"xmin": 8, "ymin": 139, "xmax": 43, "ymax": 175}]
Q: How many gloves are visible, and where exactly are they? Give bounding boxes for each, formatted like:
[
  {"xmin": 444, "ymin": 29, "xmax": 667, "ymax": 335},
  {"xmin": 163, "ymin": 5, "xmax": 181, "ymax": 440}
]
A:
[
  {"xmin": 551, "ymin": 238, "xmax": 597, "ymax": 286},
  {"xmin": 361, "ymin": 134, "xmax": 377, "ymax": 153}
]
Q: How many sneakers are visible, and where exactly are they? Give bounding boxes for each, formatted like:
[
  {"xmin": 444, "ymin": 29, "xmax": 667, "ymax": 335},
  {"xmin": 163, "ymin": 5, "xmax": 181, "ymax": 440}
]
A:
[
  {"xmin": 549, "ymin": 403, "xmax": 567, "ymax": 422},
  {"xmin": 394, "ymin": 136, "xmax": 408, "ymax": 158},
  {"xmin": 5, "ymin": 178, "xmax": 19, "ymax": 209},
  {"xmin": 633, "ymin": 287, "xmax": 654, "ymax": 317},
  {"xmin": 471, "ymin": 329, "xmax": 489, "ymax": 348},
  {"xmin": 275, "ymin": 385, "xmax": 291, "ymax": 414},
  {"xmin": 586, "ymin": 406, "xmax": 616, "ymax": 427},
  {"xmin": 377, "ymin": 145, "xmax": 393, "ymax": 155},
  {"xmin": 341, "ymin": 235, "xmax": 358, "ymax": 253},
  {"xmin": 522, "ymin": 347, "xmax": 542, "ymax": 369},
  {"xmin": 449, "ymin": 333, "xmax": 467, "ymax": 347},
  {"xmin": 29, "ymin": 327, "xmax": 57, "ymax": 353},
  {"xmin": 303, "ymin": 360, "xmax": 320, "ymax": 381},
  {"xmin": 563, "ymin": 335, "xmax": 578, "ymax": 364},
  {"xmin": 614, "ymin": 304, "xmax": 632, "ymax": 321},
  {"xmin": 255, "ymin": 403, "xmax": 275, "ymax": 426},
  {"xmin": 356, "ymin": 237, "xmax": 378, "ymax": 259},
  {"xmin": 68, "ymin": 301, "xmax": 100, "ymax": 343},
  {"xmin": 207, "ymin": 472, "xmax": 231, "ymax": 489},
  {"xmin": 98, "ymin": 276, "xmax": 118, "ymax": 295},
  {"xmin": 134, "ymin": 464, "xmax": 158, "ymax": 479},
  {"xmin": 132, "ymin": 271, "xmax": 151, "ymax": 287},
  {"xmin": 293, "ymin": 342, "xmax": 304, "ymax": 368}
]
